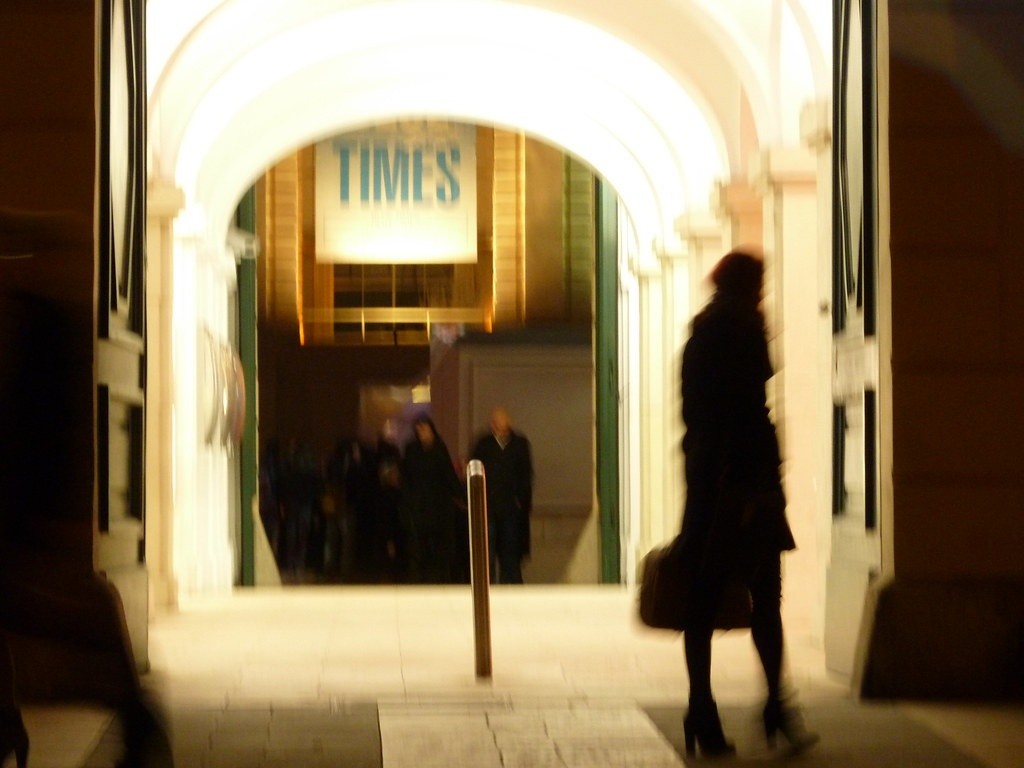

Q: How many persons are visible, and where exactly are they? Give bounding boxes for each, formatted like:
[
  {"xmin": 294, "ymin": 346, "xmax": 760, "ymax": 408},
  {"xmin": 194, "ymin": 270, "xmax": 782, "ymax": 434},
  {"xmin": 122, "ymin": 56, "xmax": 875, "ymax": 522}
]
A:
[
  {"xmin": 267, "ymin": 406, "xmax": 534, "ymax": 585},
  {"xmin": 680, "ymin": 249, "xmax": 821, "ymax": 762}
]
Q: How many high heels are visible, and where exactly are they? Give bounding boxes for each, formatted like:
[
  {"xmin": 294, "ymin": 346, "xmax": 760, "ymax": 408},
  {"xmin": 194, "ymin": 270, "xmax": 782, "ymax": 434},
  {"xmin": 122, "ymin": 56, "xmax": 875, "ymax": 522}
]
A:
[
  {"xmin": 765, "ymin": 704, "xmax": 821, "ymax": 748},
  {"xmin": 683, "ymin": 700, "xmax": 736, "ymax": 758}
]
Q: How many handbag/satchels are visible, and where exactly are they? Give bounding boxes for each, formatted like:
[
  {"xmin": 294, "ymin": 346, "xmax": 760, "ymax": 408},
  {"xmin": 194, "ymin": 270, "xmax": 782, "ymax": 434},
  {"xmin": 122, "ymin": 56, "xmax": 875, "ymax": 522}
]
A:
[{"xmin": 641, "ymin": 535, "xmax": 755, "ymax": 629}]
